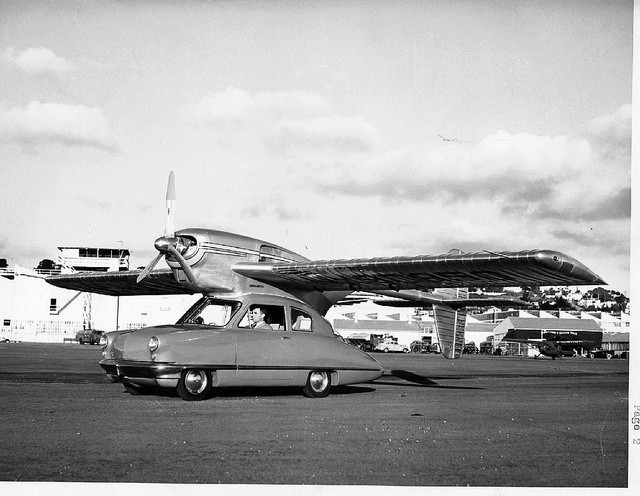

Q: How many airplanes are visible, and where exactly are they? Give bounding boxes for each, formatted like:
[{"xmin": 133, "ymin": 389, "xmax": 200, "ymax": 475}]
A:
[{"xmin": 46, "ymin": 171, "xmax": 607, "ymax": 359}]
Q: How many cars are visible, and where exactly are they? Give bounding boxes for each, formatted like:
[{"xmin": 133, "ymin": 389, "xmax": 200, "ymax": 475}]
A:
[
  {"xmin": 5, "ymin": 337, "xmax": 21, "ymax": 344},
  {"xmin": 98, "ymin": 293, "xmax": 385, "ymax": 401},
  {"xmin": 378, "ymin": 340, "xmax": 409, "ymax": 353},
  {"xmin": 587, "ymin": 347, "xmax": 614, "ymax": 359},
  {"xmin": 76, "ymin": 329, "xmax": 103, "ymax": 345}
]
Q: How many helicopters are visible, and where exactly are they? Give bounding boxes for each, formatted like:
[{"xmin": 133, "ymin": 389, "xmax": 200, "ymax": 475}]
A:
[{"xmin": 534, "ymin": 339, "xmax": 576, "ymax": 360}]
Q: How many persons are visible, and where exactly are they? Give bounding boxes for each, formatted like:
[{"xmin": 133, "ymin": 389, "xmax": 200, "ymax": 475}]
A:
[{"xmin": 248, "ymin": 306, "xmax": 271, "ymax": 332}]
